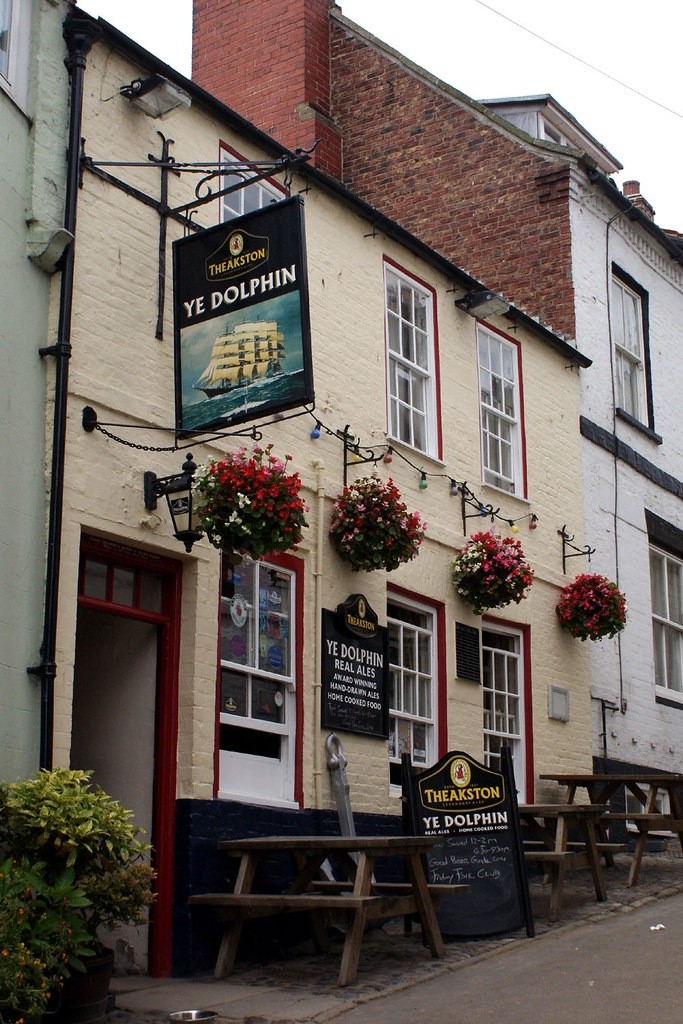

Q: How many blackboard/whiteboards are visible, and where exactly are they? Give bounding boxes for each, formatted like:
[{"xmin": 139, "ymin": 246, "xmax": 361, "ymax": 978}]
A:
[
  {"xmin": 399, "ymin": 747, "xmax": 536, "ymax": 957},
  {"xmin": 320, "ymin": 593, "xmax": 390, "ymax": 740}
]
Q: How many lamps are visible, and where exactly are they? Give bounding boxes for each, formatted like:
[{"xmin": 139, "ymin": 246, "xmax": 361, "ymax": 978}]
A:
[
  {"xmin": 118, "ymin": 73, "xmax": 192, "ymax": 122},
  {"xmin": 454, "ymin": 291, "xmax": 509, "ymax": 322},
  {"xmin": 143, "ymin": 453, "xmax": 205, "ymax": 554}
]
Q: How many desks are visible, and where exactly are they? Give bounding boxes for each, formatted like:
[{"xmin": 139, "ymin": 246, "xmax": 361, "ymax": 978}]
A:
[
  {"xmin": 518, "ymin": 803, "xmax": 608, "ymax": 921},
  {"xmin": 540, "ymin": 774, "xmax": 683, "ymax": 888},
  {"xmin": 213, "ymin": 834, "xmax": 446, "ymax": 987}
]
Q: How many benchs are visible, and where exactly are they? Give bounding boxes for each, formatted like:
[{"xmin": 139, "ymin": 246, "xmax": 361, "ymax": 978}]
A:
[
  {"xmin": 531, "ymin": 812, "xmax": 673, "ymax": 823},
  {"xmin": 523, "ymin": 851, "xmax": 574, "ymax": 864},
  {"xmin": 188, "ymin": 892, "xmax": 382, "ymax": 913},
  {"xmin": 522, "ymin": 839, "xmax": 627, "ymax": 852},
  {"xmin": 307, "ymin": 880, "xmax": 472, "ymax": 897}
]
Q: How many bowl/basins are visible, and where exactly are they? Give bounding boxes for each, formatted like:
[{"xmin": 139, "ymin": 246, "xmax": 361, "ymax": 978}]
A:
[{"xmin": 168, "ymin": 1009, "xmax": 219, "ymax": 1024}]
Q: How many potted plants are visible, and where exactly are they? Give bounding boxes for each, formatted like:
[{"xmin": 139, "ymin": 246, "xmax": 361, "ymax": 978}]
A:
[{"xmin": 0, "ymin": 767, "xmax": 159, "ymax": 1020}]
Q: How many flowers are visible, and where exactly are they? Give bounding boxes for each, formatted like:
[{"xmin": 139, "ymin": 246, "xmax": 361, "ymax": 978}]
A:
[
  {"xmin": 329, "ymin": 473, "xmax": 430, "ymax": 572},
  {"xmin": 191, "ymin": 442, "xmax": 310, "ymax": 561},
  {"xmin": 0, "ymin": 857, "xmax": 99, "ymax": 1024},
  {"xmin": 450, "ymin": 523, "xmax": 534, "ymax": 607},
  {"xmin": 555, "ymin": 572, "xmax": 628, "ymax": 641}
]
